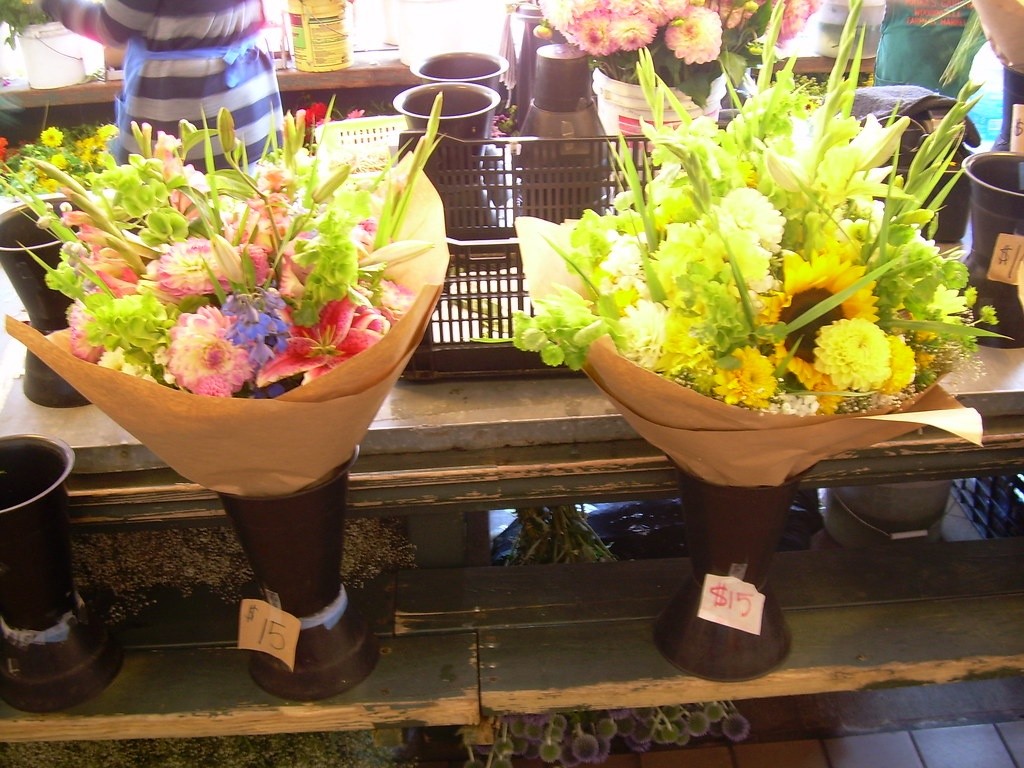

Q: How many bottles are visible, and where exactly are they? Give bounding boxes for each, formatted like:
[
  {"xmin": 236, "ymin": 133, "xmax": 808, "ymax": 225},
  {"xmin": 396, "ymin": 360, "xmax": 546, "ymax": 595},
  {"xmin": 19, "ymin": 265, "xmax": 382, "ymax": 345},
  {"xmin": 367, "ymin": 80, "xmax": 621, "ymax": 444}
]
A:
[
  {"xmin": 961, "ymin": 152, "xmax": 1024, "ymax": 348},
  {"xmin": 0, "ymin": 435, "xmax": 121, "ymax": 713},
  {"xmin": 393, "ymin": 82, "xmax": 498, "ymax": 228},
  {"xmin": 513, "ymin": 44, "xmax": 611, "ymax": 220},
  {"xmin": 410, "ymin": 52, "xmax": 510, "ymax": 206},
  {"xmin": 0, "ymin": 196, "xmax": 91, "ymax": 407}
]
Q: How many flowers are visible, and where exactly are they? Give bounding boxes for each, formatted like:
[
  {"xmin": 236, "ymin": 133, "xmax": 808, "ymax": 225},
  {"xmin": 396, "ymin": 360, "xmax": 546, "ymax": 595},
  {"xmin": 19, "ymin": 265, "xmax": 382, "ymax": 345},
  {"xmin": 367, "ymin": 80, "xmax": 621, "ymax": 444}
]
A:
[
  {"xmin": 0, "ymin": 0, "xmax": 56, "ymax": 50},
  {"xmin": 514, "ymin": 0, "xmax": 1014, "ymax": 489},
  {"xmin": 535, "ymin": 0, "xmax": 822, "ymax": 110},
  {"xmin": 1, "ymin": 90, "xmax": 451, "ymax": 496},
  {"xmin": 0, "ymin": 514, "xmax": 425, "ymax": 768},
  {"xmin": 456, "ymin": 505, "xmax": 750, "ymax": 768}
]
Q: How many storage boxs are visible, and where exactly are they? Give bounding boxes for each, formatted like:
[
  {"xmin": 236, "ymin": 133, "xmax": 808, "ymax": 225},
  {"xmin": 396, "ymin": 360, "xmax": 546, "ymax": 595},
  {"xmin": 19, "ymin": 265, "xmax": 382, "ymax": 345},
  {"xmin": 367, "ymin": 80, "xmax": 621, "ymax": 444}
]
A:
[
  {"xmin": 953, "ymin": 471, "xmax": 1024, "ymax": 539},
  {"xmin": 396, "ymin": 129, "xmax": 683, "ymax": 382}
]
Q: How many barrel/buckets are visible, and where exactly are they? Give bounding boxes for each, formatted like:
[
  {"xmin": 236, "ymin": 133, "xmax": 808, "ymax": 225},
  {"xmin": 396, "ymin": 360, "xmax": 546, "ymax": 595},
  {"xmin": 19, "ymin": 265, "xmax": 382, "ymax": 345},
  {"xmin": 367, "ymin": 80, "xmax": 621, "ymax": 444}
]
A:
[
  {"xmin": 818, "ymin": 479, "xmax": 962, "ymax": 547},
  {"xmin": 11, "ymin": 21, "xmax": 105, "ymax": 90},
  {"xmin": 283, "ymin": 0, "xmax": 354, "ymax": 73}
]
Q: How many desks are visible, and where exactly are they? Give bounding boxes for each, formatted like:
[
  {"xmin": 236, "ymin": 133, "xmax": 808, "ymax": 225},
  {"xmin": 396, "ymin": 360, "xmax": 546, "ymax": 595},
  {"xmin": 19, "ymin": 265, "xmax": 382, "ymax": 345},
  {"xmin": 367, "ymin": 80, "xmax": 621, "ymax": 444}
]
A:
[
  {"xmin": 0, "ymin": 46, "xmax": 421, "ymax": 108},
  {"xmin": 760, "ymin": 27, "xmax": 877, "ymax": 73}
]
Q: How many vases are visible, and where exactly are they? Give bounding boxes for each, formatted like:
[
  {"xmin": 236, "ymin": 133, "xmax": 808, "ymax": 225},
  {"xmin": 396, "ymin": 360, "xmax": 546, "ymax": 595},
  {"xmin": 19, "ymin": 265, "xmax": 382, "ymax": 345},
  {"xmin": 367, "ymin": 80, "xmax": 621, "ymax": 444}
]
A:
[
  {"xmin": 947, "ymin": 153, "xmax": 1024, "ymax": 349},
  {"xmin": 1, "ymin": 434, "xmax": 124, "ymax": 714},
  {"xmin": 657, "ymin": 466, "xmax": 819, "ymax": 684},
  {"xmin": 920, "ymin": 149, "xmax": 970, "ymax": 243},
  {"xmin": 1, "ymin": 198, "xmax": 92, "ymax": 409},
  {"xmin": 392, "ymin": 50, "xmax": 510, "ymax": 229},
  {"xmin": 989, "ymin": 65, "xmax": 1024, "ymax": 152},
  {"xmin": 809, "ymin": 479, "xmax": 954, "ymax": 550},
  {"xmin": 222, "ymin": 446, "xmax": 380, "ymax": 701}
]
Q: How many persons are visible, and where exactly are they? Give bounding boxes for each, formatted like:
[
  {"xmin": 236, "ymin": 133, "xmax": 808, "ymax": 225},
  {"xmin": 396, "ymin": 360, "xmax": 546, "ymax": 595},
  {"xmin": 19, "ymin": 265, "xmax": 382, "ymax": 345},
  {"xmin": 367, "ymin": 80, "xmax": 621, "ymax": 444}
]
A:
[
  {"xmin": 873, "ymin": 0, "xmax": 1024, "ymax": 151},
  {"xmin": 38, "ymin": 0, "xmax": 284, "ymax": 176}
]
